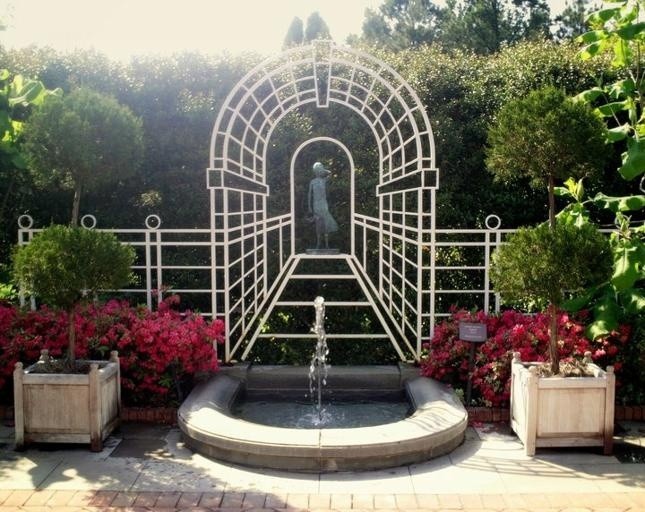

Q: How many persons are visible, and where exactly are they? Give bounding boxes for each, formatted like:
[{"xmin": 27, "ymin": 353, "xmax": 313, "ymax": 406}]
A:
[{"xmin": 307, "ymin": 162, "xmax": 339, "ymax": 249}]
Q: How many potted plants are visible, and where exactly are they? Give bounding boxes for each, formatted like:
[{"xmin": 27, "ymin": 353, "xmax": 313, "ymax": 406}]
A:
[
  {"xmin": 13, "ymin": 87, "xmax": 146, "ymax": 454},
  {"xmin": 483, "ymin": 82, "xmax": 620, "ymax": 459}
]
